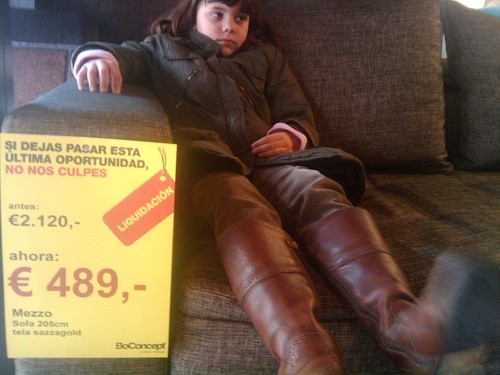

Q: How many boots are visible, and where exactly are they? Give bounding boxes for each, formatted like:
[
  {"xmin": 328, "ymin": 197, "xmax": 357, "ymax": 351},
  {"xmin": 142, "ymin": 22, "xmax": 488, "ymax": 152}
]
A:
[
  {"xmin": 215, "ymin": 218, "xmax": 344, "ymax": 375},
  {"xmin": 302, "ymin": 206, "xmax": 498, "ymax": 375}
]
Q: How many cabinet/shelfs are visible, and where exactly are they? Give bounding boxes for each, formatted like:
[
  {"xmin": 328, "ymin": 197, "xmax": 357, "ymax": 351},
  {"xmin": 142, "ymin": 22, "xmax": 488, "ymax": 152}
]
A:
[{"xmin": 12, "ymin": 38, "xmax": 82, "ymax": 110}]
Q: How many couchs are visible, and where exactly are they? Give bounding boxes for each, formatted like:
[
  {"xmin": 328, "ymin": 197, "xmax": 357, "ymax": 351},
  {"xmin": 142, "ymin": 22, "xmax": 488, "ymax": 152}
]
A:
[{"xmin": 2, "ymin": 2, "xmax": 500, "ymax": 374}]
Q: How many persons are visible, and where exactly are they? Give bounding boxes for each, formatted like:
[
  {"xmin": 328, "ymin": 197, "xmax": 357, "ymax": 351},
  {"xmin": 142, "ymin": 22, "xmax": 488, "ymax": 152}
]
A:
[{"xmin": 71, "ymin": 0, "xmax": 500, "ymax": 375}]
